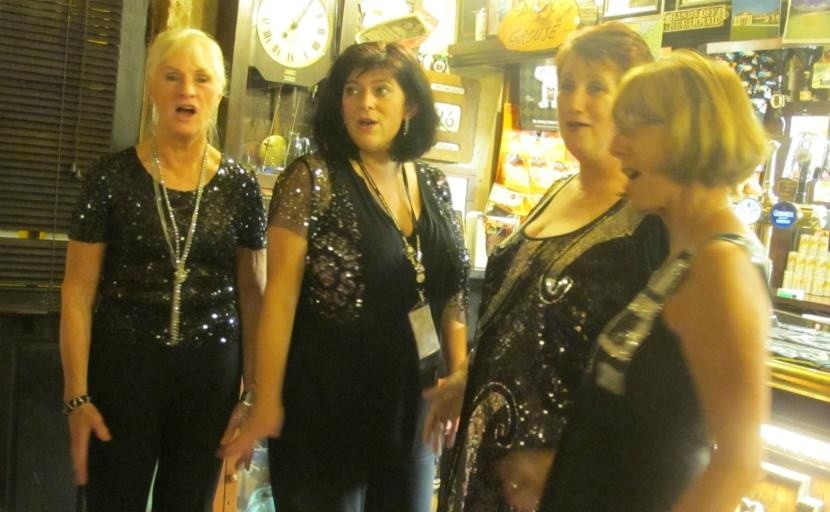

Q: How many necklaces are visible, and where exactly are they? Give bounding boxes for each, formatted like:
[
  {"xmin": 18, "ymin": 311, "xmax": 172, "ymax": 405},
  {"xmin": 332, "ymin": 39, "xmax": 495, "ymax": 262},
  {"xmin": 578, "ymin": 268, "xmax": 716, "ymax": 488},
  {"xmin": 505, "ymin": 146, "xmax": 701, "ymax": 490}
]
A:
[{"xmin": 149, "ymin": 136, "xmax": 210, "ymax": 347}]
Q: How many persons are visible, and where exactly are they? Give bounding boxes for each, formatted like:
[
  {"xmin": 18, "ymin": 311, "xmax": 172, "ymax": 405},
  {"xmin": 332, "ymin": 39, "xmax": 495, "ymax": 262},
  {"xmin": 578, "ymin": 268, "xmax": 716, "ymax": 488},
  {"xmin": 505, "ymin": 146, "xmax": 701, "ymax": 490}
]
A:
[
  {"xmin": 56, "ymin": 25, "xmax": 267, "ymax": 511},
  {"xmin": 212, "ymin": 41, "xmax": 470, "ymax": 512},
  {"xmin": 436, "ymin": 19, "xmax": 671, "ymax": 512},
  {"xmin": 535, "ymin": 46, "xmax": 775, "ymax": 512}
]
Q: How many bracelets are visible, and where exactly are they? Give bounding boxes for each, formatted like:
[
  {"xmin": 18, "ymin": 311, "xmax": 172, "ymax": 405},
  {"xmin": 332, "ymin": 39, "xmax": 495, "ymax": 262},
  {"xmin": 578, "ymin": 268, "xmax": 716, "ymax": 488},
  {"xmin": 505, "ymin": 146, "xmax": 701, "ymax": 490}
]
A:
[
  {"xmin": 62, "ymin": 394, "xmax": 91, "ymax": 417},
  {"xmin": 240, "ymin": 390, "xmax": 255, "ymax": 406}
]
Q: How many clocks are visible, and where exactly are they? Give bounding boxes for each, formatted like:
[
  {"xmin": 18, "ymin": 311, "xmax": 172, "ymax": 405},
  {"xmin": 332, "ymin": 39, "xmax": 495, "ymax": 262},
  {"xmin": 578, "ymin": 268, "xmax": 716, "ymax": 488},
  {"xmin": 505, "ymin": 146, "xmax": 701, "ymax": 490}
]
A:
[{"xmin": 223, "ymin": 0, "xmax": 356, "ymax": 190}]
